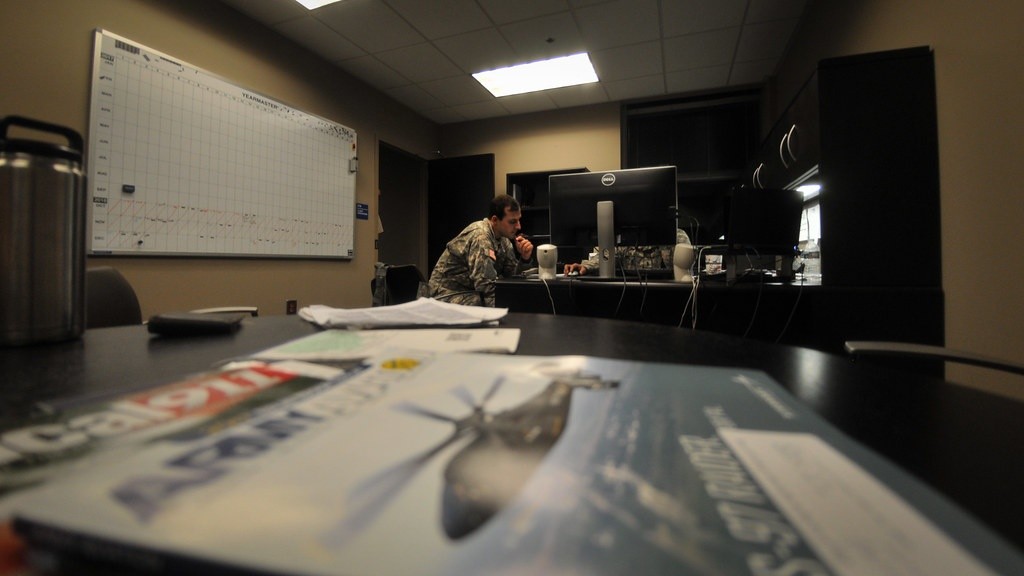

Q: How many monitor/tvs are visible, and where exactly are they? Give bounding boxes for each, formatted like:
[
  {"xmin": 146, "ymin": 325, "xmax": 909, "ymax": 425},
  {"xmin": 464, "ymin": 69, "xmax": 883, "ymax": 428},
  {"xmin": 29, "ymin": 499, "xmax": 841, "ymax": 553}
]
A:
[
  {"xmin": 728, "ymin": 186, "xmax": 802, "ymax": 256},
  {"xmin": 549, "ymin": 166, "xmax": 678, "ymax": 281}
]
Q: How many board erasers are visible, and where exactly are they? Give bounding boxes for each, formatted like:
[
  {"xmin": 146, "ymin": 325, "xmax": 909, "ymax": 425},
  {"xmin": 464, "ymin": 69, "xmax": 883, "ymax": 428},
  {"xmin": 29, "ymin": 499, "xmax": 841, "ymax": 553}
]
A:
[{"xmin": 122, "ymin": 185, "xmax": 135, "ymax": 193}]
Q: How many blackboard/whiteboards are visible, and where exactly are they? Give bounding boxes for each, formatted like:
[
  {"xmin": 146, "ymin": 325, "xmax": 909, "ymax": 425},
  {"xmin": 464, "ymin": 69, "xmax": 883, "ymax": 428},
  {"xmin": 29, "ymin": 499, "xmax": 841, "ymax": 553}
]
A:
[{"xmin": 83, "ymin": 27, "xmax": 358, "ymax": 260}]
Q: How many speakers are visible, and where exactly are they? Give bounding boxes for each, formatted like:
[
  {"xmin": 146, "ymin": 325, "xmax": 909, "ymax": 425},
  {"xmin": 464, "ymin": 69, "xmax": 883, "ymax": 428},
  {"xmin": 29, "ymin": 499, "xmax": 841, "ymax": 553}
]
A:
[
  {"xmin": 537, "ymin": 245, "xmax": 559, "ymax": 280},
  {"xmin": 673, "ymin": 244, "xmax": 696, "ymax": 283}
]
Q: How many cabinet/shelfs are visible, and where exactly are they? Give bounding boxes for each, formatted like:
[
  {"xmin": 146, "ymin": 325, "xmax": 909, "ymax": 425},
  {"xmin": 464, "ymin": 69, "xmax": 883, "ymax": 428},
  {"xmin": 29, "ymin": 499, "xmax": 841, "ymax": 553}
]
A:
[
  {"xmin": 729, "ymin": 44, "xmax": 946, "ymax": 381},
  {"xmin": 506, "ymin": 168, "xmax": 590, "ymax": 264}
]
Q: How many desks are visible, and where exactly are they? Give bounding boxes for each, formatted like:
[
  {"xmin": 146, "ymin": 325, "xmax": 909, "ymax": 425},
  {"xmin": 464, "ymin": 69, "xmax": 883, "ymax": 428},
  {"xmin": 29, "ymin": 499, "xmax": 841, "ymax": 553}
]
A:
[
  {"xmin": 1, "ymin": 307, "xmax": 1023, "ymax": 575},
  {"xmin": 492, "ymin": 278, "xmax": 822, "ymax": 332}
]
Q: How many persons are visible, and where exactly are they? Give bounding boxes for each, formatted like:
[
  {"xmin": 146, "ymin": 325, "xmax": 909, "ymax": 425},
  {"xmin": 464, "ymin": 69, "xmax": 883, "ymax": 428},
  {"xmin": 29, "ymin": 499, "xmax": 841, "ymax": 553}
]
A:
[
  {"xmin": 417, "ymin": 194, "xmax": 533, "ymax": 306},
  {"xmin": 564, "ymin": 229, "xmax": 691, "ymax": 275}
]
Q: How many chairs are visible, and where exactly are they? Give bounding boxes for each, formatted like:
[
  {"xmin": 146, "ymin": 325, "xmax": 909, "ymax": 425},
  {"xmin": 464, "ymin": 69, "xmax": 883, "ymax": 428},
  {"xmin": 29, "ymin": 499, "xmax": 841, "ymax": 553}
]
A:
[{"xmin": 384, "ymin": 264, "xmax": 426, "ymax": 304}]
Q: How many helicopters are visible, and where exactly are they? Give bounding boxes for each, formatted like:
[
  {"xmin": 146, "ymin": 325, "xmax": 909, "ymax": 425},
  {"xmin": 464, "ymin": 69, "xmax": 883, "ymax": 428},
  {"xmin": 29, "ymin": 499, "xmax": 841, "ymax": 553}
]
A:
[{"xmin": 345, "ymin": 362, "xmax": 621, "ymax": 547}]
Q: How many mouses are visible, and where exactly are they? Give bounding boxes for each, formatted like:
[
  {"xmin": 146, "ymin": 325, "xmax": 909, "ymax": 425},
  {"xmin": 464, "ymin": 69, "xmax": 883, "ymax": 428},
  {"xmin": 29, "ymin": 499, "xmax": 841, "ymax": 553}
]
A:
[{"xmin": 567, "ymin": 271, "xmax": 579, "ymax": 276}]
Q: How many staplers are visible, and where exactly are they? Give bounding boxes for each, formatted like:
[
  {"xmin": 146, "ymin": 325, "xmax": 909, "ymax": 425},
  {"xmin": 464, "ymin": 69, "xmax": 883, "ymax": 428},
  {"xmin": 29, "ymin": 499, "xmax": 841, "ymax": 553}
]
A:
[{"xmin": 521, "ymin": 268, "xmax": 538, "ymax": 279}]
